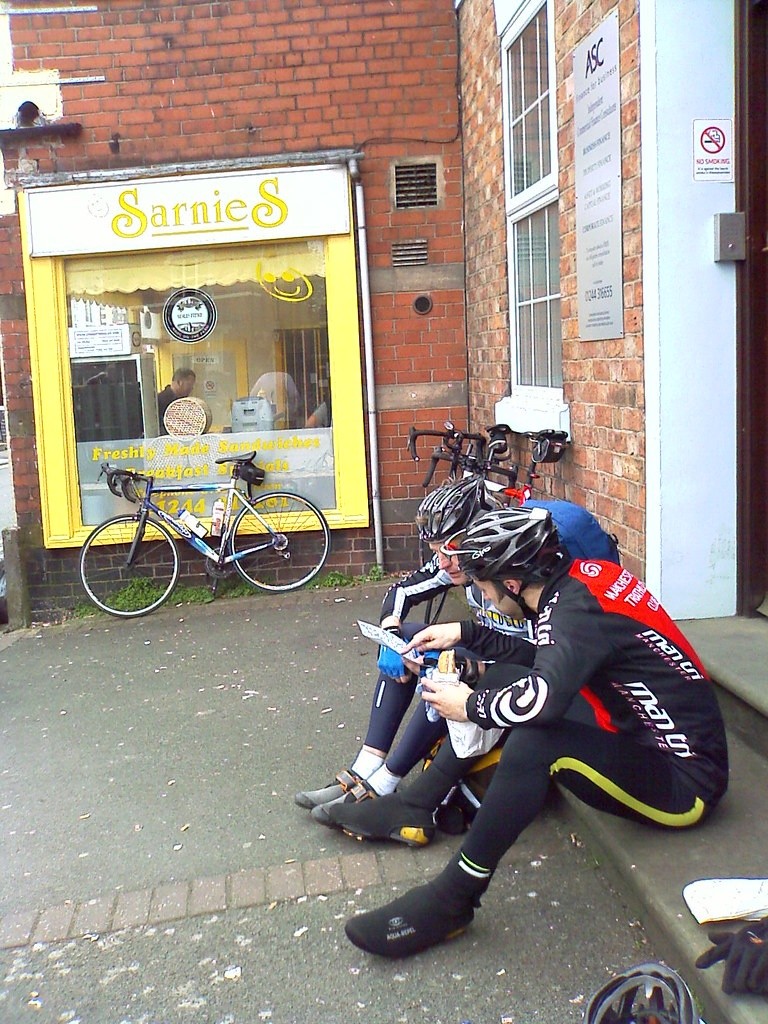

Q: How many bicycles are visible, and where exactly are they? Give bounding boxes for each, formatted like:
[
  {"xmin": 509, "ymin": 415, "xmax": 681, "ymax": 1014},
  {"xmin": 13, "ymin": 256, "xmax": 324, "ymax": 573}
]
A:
[
  {"xmin": 404, "ymin": 424, "xmax": 570, "ymax": 627},
  {"xmin": 80, "ymin": 451, "xmax": 332, "ymax": 620}
]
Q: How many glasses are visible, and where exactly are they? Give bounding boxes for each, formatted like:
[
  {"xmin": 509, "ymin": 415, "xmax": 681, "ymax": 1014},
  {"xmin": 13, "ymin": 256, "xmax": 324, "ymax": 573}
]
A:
[{"xmin": 439, "ymin": 529, "xmax": 480, "ymax": 560}]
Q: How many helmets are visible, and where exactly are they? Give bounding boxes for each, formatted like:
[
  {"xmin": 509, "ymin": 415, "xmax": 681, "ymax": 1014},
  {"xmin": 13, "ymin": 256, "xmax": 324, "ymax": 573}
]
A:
[
  {"xmin": 415, "ymin": 474, "xmax": 491, "ymax": 542},
  {"xmin": 459, "ymin": 506, "xmax": 564, "ymax": 582},
  {"xmin": 584, "ymin": 963, "xmax": 700, "ymax": 1023}
]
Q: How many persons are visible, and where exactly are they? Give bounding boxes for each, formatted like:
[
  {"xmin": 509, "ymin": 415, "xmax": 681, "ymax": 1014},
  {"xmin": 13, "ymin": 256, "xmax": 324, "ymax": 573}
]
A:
[
  {"xmin": 152, "ymin": 366, "xmax": 197, "ymax": 437},
  {"xmin": 292, "ymin": 476, "xmax": 555, "ymax": 827},
  {"xmin": 325, "ymin": 508, "xmax": 730, "ymax": 955},
  {"xmin": 304, "ymin": 378, "xmax": 332, "ymax": 428}
]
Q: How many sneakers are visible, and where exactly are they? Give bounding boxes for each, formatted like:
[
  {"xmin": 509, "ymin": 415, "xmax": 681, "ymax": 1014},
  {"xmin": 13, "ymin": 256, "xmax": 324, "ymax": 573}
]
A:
[
  {"xmin": 295, "ymin": 769, "xmax": 360, "ymax": 807},
  {"xmin": 310, "ymin": 779, "xmax": 397, "ymax": 827}
]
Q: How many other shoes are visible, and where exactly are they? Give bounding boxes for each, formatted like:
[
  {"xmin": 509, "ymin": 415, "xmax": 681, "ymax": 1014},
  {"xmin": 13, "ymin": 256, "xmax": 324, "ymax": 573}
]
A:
[
  {"xmin": 328, "ymin": 792, "xmax": 434, "ymax": 847},
  {"xmin": 344, "ymin": 882, "xmax": 475, "ymax": 956}
]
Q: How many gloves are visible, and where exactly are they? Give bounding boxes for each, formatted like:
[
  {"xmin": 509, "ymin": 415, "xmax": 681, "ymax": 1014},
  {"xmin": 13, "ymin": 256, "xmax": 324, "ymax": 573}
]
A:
[{"xmin": 695, "ymin": 915, "xmax": 768, "ymax": 996}]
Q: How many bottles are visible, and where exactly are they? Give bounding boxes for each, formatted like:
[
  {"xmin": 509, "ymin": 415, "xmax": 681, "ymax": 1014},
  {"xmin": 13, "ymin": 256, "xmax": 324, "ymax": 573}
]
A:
[
  {"xmin": 211, "ymin": 498, "xmax": 225, "ymax": 537},
  {"xmin": 178, "ymin": 510, "xmax": 209, "ymax": 539}
]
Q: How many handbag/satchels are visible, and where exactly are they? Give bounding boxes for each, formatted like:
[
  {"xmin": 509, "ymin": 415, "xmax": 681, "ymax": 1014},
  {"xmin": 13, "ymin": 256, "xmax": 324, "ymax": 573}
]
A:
[
  {"xmin": 422, "ymin": 732, "xmax": 503, "ymax": 835},
  {"xmin": 521, "ymin": 500, "xmax": 621, "ymax": 568}
]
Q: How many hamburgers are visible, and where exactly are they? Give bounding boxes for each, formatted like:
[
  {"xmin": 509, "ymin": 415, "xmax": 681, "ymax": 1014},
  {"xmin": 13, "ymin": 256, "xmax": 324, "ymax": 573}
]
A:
[{"xmin": 438, "ymin": 649, "xmax": 456, "ymax": 673}]
